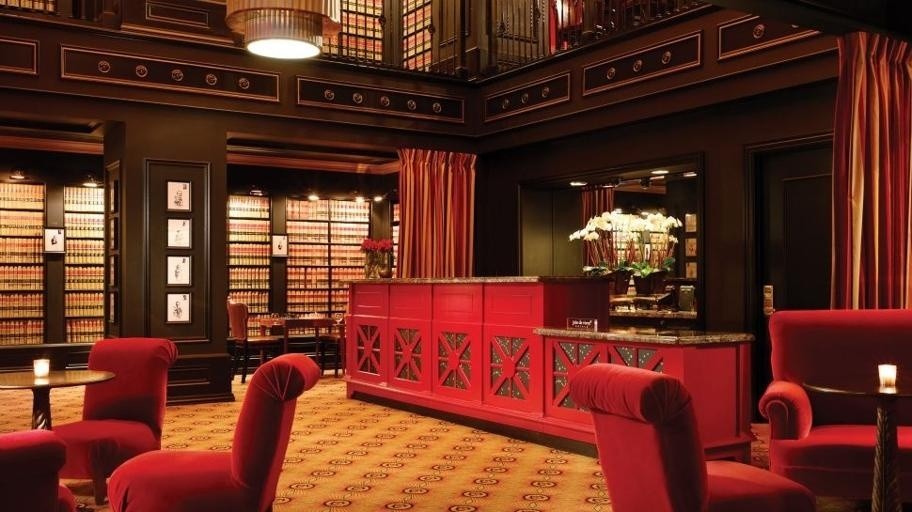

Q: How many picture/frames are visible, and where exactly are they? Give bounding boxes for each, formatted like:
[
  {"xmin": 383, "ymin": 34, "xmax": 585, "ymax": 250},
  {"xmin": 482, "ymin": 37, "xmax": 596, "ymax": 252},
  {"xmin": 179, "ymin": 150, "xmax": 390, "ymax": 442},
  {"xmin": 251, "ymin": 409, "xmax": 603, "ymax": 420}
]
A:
[
  {"xmin": 161, "ymin": 178, "xmax": 194, "ymax": 325},
  {"xmin": 42, "ymin": 226, "xmax": 67, "ymax": 254},
  {"xmin": 684, "ymin": 210, "xmax": 701, "ymax": 281},
  {"xmin": 269, "ymin": 233, "xmax": 290, "ymax": 258}
]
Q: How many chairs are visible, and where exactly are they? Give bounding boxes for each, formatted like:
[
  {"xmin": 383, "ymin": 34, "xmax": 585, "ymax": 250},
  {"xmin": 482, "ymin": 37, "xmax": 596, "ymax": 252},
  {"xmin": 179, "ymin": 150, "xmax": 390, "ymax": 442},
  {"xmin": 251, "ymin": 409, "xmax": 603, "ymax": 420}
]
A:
[
  {"xmin": 318, "ymin": 314, "xmax": 347, "ymax": 378},
  {"xmin": 226, "ymin": 299, "xmax": 285, "ymax": 384},
  {"xmin": 1, "ymin": 428, "xmax": 78, "ymax": 511},
  {"xmin": 51, "ymin": 339, "xmax": 180, "ymax": 505},
  {"xmin": 568, "ymin": 361, "xmax": 820, "ymax": 512},
  {"xmin": 105, "ymin": 352, "xmax": 319, "ymax": 510},
  {"xmin": 756, "ymin": 308, "xmax": 912, "ymax": 499}
]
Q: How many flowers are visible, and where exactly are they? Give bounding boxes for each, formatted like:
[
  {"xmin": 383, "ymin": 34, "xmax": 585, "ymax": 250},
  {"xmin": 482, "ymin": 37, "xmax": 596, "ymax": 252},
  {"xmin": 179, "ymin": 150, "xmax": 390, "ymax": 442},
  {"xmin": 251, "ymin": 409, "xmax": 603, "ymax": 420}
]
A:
[
  {"xmin": 566, "ymin": 206, "xmax": 632, "ymax": 280},
  {"xmin": 629, "ymin": 211, "xmax": 684, "ymax": 278},
  {"xmin": 359, "ymin": 238, "xmax": 394, "ymax": 252}
]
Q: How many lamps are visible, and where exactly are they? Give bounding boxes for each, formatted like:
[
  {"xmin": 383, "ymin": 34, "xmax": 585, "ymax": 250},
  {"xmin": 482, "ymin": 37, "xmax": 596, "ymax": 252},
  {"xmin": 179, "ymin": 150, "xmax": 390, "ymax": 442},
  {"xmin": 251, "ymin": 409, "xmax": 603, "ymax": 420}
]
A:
[
  {"xmin": 225, "ymin": 0, "xmax": 343, "ymax": 61},
  {"xmin": 33, "ymin": 358, "xmax": 51, "ymax": 378}
]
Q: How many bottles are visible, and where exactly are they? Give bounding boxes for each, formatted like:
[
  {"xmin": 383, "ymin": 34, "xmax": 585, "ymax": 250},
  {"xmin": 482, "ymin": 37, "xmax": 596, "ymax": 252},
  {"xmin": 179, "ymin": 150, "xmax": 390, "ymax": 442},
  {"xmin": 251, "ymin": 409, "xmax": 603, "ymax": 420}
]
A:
[
  {"xmin": 0, "ymin": 183, "xmax": 44, "ymax": 345},
  {"xmin": 65, "ymin": 186, "xmax": 105, "ymax": 344},
  {"xmin": 392, "ymin": 203, "xmax": 402, "ymax": 278},
  {"xmin": 227, "ymin": 196, "xmax": 273, "ymax": 340},
  {"xmin": 285, "ymin": 198, "xmax": 373, "ymax": 334},
  {"xmin": 269, "ymin": 312, "xmax": 288, "ymax": 325}
]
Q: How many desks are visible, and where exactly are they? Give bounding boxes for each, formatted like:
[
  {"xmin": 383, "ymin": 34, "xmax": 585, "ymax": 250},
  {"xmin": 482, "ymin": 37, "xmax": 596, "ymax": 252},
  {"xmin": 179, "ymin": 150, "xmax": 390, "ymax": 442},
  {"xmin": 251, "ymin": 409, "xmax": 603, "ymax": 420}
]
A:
[
  {"xmin": 802, "ymin": 374, "xmax": 911, "ymax": 511},
  {"xmin": 258, "ymin": 317, "xmax": 337, "ymax": 363},
  {"xmin": 1, "ymin": 371, "xmax": 118, "ymax": 430}
]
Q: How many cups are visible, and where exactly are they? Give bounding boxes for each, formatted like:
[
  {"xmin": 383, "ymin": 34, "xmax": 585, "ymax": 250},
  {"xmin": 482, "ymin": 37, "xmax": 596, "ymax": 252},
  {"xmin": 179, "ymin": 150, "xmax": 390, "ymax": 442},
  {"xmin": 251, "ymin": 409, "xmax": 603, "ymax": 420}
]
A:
[
  {"xmin": 33, "ymin": 378, "xmax": 50, "ymax": 385},
  {"xmin": 33, "ymin": 359, "xmax": 50, "ymax": 377},
  {"xmin": 879, "ymin": 387, "xmax": 896, "ymax": 393}
]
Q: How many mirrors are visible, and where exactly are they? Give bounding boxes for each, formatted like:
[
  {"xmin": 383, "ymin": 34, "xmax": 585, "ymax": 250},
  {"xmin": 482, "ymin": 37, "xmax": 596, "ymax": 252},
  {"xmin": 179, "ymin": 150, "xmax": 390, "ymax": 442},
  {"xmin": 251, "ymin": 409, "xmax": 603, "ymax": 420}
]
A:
[{"xmin": 514, "ymin": 150, "xmax": 707, "ymax": 285}]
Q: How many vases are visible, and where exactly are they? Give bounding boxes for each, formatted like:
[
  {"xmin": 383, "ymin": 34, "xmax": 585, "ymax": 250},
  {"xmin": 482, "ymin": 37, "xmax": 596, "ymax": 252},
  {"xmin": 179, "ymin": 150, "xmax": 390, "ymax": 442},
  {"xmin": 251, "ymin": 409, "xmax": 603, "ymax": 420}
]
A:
[
  {"xmin": 379, "ymin": 252, "xmax": 391, "ymax": 278},
  {"xmin": 367, "ymin": 253, "xmax": 379, "ymax": 279},
  {"xmin": 632, "ymin": 271, "xmax": 665, "ymax": 294},
  {"xmin": 595, "ymin": 270, "xmax": 631, "ymax": 295}
]
